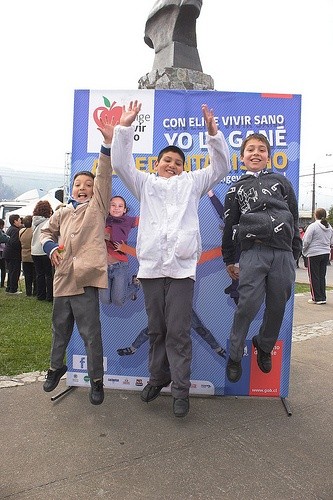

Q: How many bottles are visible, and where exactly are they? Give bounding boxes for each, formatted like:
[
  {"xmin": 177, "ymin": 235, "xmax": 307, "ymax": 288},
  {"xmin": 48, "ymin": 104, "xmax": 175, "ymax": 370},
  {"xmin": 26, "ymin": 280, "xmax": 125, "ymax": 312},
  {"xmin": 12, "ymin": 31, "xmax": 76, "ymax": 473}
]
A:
[{"xmin": 58, "ymin": 245, "xmax": 65, "ymax": 253}]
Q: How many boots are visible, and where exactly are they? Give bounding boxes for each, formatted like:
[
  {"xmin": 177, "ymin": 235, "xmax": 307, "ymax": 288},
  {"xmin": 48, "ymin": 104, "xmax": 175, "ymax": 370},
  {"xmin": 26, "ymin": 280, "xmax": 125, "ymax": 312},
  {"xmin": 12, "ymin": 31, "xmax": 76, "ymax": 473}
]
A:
[{"xmin": 117, "ymin": 348, "xmax": 134, "ymax": 356}]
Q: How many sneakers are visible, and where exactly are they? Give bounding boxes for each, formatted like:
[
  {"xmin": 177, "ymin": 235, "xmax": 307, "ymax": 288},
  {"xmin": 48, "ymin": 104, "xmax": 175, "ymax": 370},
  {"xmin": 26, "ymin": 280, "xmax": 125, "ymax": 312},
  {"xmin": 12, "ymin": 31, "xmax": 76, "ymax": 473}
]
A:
[
  {"xmin": 43, "ymin": 365, "xmax": 68, "ymax": 392},
  {"xmin": 218, "ymin": 348, "xmax": 229, "ymax": 360},
  {"xmin": 141, "ymin": 378, "xmax": 171, "ymax": 402},
  {"xmin": 173, "ymin": 396, "xmax": 190, "ymax": 417},
  {"xmin": 26, "ymin": 291, "xmax": 53, "ymax": 302},
  {"xmin": 89, "ymin": 376, "xmax": 104, "ymax": 405},
  {"xmin": 253, "ymin": 336, "xmax": 272, "ymax": 373},
  {"xmin": 307, "ymin": 298, "xmax": 327, "ymax": 305},
  {"xmin": 6, "ymin": 288, "xmax": 23, "ymax": 295},
  {"xmin": 226, "ymin": 354, "xmax": 242, "ymax": 383},
  {"xmin": 131, "ymin": 276, "xmax": 139, "ymax": 301}
]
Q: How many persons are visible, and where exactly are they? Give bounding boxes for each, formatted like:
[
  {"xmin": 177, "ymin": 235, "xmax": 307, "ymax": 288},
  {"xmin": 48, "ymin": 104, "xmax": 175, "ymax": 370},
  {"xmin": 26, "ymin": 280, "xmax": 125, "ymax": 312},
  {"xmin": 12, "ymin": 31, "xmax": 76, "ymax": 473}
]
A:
[
  {"xmin": 111, "ymin": 99, "xmax": 231, "ymax": 417},
  {"xmin": 31, "ymin": 200, "xmax": 53, "ymax": 301},
  {"xmin": 221, "ymin": 134, "xmax": 303, "ymax": 383},
  {"xmin": 99, "ymin": 196, "xmax": 139, "ymax": 307},
  {"xmin": 43, "ymin": 114, "xmax": 118, "ymax": 405},
  {"xmin": 0, "ymin": 219, "xmax": 10, "ymax": 288},
  {"xmin": 296, "ymin": 226, "xmax": 308, "ymax": 270},
  {"xmin": 6, "ymin": 214, "xmax": 23, "ymax": 294},
  {"xmin": 111, "ymin": 240, "xmax": 229, "ymax": 361},
  {"xmin": 302, "ymin": 208, "xmax": 333, "ymax": 304},
  {"xmin": 19, "ymin": 215, "xmax": 37, "ymax": 296}
]
